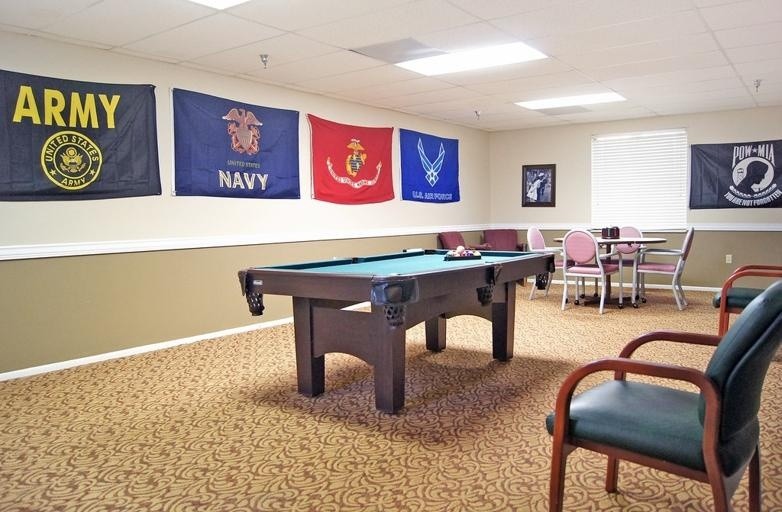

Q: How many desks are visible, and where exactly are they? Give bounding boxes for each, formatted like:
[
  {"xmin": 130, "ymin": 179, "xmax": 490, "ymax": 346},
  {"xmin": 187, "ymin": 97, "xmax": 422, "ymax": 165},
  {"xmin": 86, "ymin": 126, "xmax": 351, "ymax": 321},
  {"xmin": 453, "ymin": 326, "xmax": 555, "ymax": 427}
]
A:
[
  {"xmin": 552, "ymin": 236, "xmax": 668, "ymax": 306},
  {"xmin": 238, "ymin": 248, "xmax": 557, "ymax": 416}
]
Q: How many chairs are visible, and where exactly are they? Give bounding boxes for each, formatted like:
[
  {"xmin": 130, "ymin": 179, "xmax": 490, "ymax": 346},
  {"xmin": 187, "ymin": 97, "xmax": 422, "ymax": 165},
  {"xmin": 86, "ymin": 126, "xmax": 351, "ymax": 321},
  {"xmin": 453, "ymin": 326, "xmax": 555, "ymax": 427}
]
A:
[
  {"xmin": 562, "ymin": 225, "xmax": 695, "ymax": 315},
  {"xmin": 438, "ymin": 226, "xmax": 575, "ymax": 300},
  {"xmin": 545, "ymin": 279, "xmax": 780, "ymax": 512},
  {"xmin": 712, "ymin": 264, "xmax": 782, "ymax": 352}
]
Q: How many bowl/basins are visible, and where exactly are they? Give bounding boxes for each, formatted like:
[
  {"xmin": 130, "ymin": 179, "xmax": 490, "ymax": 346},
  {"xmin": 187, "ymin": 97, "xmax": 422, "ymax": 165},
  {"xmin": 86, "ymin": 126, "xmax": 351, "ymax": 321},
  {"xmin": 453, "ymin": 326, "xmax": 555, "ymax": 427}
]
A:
[{"xmin": 601, "ymin": 228, "xmax": 619, "ymax": 240}]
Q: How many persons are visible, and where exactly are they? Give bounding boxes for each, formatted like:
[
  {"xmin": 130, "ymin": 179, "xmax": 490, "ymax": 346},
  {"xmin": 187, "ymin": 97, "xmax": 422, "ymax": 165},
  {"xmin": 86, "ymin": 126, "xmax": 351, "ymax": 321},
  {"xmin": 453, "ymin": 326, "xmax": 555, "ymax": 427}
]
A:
[{"xmin": 738, "ymin": 161, "xmax": 768, "ymax": 193}]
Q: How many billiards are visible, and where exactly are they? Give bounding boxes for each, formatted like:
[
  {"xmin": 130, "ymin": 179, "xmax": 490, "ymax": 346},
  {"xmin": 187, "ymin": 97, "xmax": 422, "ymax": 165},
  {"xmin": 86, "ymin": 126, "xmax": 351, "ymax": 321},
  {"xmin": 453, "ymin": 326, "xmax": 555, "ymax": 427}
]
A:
[{"xmin": 446, "ymin": 246, "xmax": 481, "ymax": 257}]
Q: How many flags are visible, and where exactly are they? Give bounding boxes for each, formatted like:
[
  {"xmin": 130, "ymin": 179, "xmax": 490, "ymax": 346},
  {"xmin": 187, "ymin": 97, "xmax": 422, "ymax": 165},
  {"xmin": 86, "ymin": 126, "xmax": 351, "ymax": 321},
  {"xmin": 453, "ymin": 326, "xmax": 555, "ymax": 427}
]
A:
[
  {"xmin": 690, "ymin": 140, "xmax": 782, "ymax": 209},
  {"xmin": 399, "ymin": 129, "xmax": 460, "ymax": 203},
  {"xmin": 170, "ymin": 87, "xmax": 300, "ymax": 199},
  {"xmin": 0, "ymin": 68, "xmax": 161, "ymax": 201},
  {"xmin": 305, "ymin": 113, "xmax": 395, "ymax": 204}
]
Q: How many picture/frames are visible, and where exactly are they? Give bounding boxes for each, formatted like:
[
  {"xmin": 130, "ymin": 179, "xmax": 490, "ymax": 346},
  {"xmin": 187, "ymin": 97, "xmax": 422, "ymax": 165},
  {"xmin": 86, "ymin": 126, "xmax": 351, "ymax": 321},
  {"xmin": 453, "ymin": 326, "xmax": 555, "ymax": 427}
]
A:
[{"xmin": 521, "ymin": 164, "xmax": 556, "ymax": 208}]
